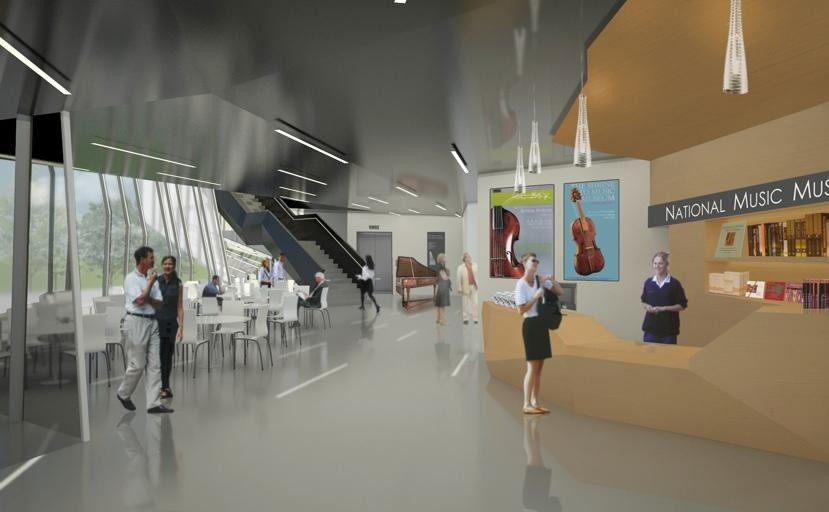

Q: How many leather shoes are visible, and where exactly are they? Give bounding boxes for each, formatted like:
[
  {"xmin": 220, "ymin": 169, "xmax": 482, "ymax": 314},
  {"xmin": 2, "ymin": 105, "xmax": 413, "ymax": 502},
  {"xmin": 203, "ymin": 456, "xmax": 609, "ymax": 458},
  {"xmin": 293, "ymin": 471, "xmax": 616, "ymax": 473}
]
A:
[
  {"xmin": 116, "ymin": 392, "xmax": 137, "ymax": 410},
  {"xmin": 146, "ymin": 404, "xmax": 173, "ymax": 413},
  {"xmin": 523, "ymin": 406, "xmax": 550, "ymax": 413}
]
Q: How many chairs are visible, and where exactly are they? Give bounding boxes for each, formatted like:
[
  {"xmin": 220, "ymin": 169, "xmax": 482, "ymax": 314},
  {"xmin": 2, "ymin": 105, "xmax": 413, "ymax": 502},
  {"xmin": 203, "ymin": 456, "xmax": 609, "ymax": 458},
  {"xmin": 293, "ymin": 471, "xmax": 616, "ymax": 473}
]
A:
[{"xmin": 0, "ymin": 274, "xmax": 333, "ymax": 392}]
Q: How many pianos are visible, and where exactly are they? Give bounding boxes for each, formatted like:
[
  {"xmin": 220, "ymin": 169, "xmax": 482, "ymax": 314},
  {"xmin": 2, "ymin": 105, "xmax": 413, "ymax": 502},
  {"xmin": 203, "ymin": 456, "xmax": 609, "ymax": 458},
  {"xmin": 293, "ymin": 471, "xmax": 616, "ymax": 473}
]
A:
[{"xmin": 396, "ymin": 256, "xmax": 439, "ymax": 311}]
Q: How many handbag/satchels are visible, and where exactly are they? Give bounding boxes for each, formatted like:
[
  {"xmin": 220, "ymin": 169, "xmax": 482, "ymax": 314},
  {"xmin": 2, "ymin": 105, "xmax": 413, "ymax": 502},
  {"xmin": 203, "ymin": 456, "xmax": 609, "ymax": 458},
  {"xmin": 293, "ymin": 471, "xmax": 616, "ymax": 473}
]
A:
[{"xmin": 537, "ymin": 287, "xmax": 562, "ymax": 328}]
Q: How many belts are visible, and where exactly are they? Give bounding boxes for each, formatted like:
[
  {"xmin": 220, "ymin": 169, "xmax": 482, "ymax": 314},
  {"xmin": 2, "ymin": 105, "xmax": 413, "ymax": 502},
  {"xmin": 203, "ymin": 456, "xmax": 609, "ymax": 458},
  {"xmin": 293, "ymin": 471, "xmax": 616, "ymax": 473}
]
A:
[{"xmin": 126, "ymin": 310, "xmax": 157, "ymax": 321}]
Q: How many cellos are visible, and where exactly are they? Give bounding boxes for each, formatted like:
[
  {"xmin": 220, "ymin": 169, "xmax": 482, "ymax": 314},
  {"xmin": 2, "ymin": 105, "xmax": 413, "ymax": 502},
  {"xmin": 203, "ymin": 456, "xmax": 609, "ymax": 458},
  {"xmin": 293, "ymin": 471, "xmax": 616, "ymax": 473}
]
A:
[{"xmin": 490, "ymin": 206, "xmax": 525, "ymax": 279}]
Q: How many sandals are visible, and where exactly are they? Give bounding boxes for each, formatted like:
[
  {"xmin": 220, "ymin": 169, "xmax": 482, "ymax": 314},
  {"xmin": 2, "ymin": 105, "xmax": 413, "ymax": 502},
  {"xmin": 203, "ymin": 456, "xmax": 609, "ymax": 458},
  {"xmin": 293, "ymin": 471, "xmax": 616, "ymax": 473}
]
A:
[{"xmin": 160, "ymin": 389, "xmax": 172, "ymax": 399}]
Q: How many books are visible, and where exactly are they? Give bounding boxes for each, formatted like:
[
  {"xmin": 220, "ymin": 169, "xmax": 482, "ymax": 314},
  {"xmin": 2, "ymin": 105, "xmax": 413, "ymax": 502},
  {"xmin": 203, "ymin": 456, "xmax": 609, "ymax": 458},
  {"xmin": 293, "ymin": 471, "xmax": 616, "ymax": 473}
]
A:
[{"xmin": 708, "ymin": 212, "xmax": 829, "ymax": 309}]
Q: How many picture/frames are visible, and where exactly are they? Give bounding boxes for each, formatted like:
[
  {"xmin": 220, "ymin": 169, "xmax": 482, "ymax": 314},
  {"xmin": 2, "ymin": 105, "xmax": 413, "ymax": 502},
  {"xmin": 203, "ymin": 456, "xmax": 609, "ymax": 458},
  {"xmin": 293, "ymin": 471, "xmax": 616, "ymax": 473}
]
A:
[
  {"xmin": 560, "ymin": 178, "xmax": 622, "ymax": 283},
  {"xmin": 488, "ymin": 183, "xmax": 557, "ymax": 284}
]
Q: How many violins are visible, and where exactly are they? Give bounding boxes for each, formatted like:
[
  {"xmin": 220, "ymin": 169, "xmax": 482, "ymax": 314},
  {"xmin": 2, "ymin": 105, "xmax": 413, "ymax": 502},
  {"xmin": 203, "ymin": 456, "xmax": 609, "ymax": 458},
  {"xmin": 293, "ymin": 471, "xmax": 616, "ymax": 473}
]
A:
[{"xmin": 570, "ymin": 187, "xmax": 605, "ymax": 276}]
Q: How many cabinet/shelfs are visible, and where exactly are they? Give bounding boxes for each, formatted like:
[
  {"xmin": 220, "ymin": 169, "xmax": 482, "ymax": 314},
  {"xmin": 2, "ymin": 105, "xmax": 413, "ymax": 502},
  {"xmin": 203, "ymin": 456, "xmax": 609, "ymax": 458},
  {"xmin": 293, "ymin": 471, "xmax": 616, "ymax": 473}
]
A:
[{"xmin": 705, "ymin": 254, "xmax": 829, "ymax": 308}]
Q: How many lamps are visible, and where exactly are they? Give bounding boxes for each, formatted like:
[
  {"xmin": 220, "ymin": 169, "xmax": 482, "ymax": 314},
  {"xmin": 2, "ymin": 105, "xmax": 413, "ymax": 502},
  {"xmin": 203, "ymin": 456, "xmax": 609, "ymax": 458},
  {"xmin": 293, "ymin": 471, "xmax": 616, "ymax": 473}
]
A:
[
  {"xmin": 715, "ymin": 0, "xmax": 756, "ymax": 97},
  {"xmin": 572, "ymin": 51, "xmax": 593, "ymax": 169},
  {"xmin": 528, "ymin": 99, "xmax": 542, "ymax": 174},
  {"xmin": 514, "ymin": 124, "xmax": 526, "ymax": 196}
]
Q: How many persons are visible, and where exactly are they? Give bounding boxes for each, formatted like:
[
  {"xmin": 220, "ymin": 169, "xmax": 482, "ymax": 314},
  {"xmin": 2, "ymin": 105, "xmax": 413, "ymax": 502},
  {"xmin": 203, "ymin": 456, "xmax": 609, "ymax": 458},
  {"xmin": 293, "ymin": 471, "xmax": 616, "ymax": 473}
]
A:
[
  {"xmin": 258, "ymin": 257, "xmax": 273, "ymax": 287},
  {"xmin": 456, "ymin": 252, "xmax": 481, "ymax": 325},
  {"xmin": 512, "ymin": 250, "xmax": 565, "ymax": 415},
  {"xmin": 432, "ymin": 251, "xmax": 453, "ymax": 327},
  {"xmin": 201, "ymin": 274, "xmax": 224, "ymax": 308},
  {"xmin": 273, "ymin": 252, "xmax": 287, "ymax": 283},
  {"xmin": 639, "ymin": 250, "xmax": 689, "ymax": 345},
  {"xmin": 156, "ymin": 254, "xmax": 187, "ymax": 401},
  {"xmin": 115, "ymin": 244, "xmax": 177, "ymax": 414},
  {"xmin": 355, "ymin": 253, "xmax": 381, "ymax": 314},
  {"xmin": 296, "ymin": 272, "xmax": 328, "ymax": 320}
]
